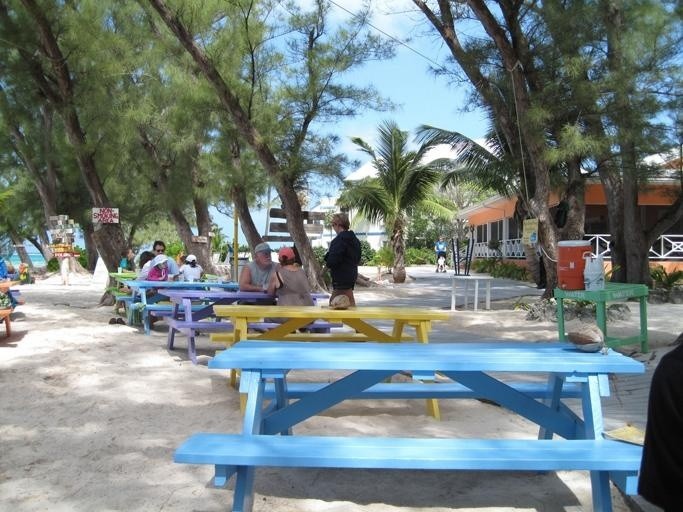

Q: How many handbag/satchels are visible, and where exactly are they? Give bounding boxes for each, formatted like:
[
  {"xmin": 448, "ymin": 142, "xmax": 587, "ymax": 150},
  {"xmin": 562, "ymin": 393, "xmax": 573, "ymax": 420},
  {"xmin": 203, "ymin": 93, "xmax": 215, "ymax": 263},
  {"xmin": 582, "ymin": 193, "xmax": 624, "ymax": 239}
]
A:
[{"xmin": 331, "ymin": 295, "xmax": 351, "ymax": 308}]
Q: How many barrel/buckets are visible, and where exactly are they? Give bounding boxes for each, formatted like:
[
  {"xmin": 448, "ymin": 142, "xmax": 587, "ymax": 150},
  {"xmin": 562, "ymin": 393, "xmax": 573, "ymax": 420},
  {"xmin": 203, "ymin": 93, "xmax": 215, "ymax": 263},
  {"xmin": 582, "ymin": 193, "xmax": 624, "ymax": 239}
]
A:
[
  {"xmin": 582, "ymin": 251, "xmax": 605, "ymax": 292},
  {"xmin": 556, "ymin": 239, "xmax": 592, "ymax": 289}
]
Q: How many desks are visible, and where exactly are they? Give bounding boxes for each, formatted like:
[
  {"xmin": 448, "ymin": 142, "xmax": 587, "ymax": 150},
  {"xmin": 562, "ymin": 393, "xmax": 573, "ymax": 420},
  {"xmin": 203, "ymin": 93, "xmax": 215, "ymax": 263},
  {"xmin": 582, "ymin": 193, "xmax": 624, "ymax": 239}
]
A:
[
  {"xmin": 206, "ymin": 339, "xmax": 645, "ymax": 512},
  {"xmin": 553, "ymin": 280, "xmax": 649, "ymax": 356},
  {"xmin": 450, "ymin": 273, "xmax": 494, "ymax": 311}
]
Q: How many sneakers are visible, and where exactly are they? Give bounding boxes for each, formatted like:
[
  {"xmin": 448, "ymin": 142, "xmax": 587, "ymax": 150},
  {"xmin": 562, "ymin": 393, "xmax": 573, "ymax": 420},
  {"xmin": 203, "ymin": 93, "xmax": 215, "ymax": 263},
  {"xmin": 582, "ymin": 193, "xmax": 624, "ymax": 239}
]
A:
[
  {"xmin": 109, "ymin": 318, "xmax": 117, "ymax": 324},
  {"xmin": 118, "ymin": 318, "xmax": 125, "ymax": 325}
]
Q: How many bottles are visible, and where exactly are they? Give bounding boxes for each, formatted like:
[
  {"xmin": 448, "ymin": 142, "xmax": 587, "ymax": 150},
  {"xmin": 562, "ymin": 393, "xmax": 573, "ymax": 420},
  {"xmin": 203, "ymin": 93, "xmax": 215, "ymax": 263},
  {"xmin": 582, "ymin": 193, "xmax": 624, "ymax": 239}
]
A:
[{"xmin": 584, "ymin": 254, "xmax": 605, "ymax": 290}]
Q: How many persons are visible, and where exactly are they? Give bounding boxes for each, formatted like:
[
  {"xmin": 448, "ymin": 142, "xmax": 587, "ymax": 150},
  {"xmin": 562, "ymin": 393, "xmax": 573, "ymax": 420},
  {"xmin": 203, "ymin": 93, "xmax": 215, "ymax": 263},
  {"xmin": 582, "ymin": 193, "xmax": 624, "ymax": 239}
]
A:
[
  {"xmin": 434, "ymin": 236, "xmax": 447, "ymax": 253},
  {"xmin": 118, "ymin": 248, "xmax": 134, "ymax": 273},
  {"xmin": 263, "ymin": 245, "xmax": 316, "ymax": 325},
  {"xmin": 436, "ymin": 255, "xmax": 445, "ymax": 272},
  {"xmin": 172, "ymin": 255, "xmax": 204, "ymax": 299},
  {"xmin": 136, "ymin": 255, "xmax": 168, "ymax": 299},
  {"xmin": 151, "ymin": 240, "xmax": 177, "ymax": 274},
  {"xmin": 322, "ymin": 212, "xmax": 362, "ymax": 308},
  {"xmin": 0, "ymin": 255, "xmax": 29, "ymax": 311},
  {"xmin": 236, "ymin": 242, "xmax": 279, "ymax": 306},
  {"xmin": 118, "ymin": 252, "xmax": 155, "ymax": 295}
]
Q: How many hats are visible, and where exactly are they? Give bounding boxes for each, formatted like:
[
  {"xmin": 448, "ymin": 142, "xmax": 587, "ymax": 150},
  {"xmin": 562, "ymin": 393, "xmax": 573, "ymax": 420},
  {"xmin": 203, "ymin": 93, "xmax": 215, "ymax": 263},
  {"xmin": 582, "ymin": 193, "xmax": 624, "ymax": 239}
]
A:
[
  {"xmin": 153, "ymin": 255, "xmax": 168, "ymax": 267},
  {"xmin": 279, "ymin": 247, "xmax": 295, "ymax": 260},
  {"xmin": 186, "ymin": 255, "xmax": 197, "ymax": 262},
  {"xmin": 255, "ymin": 244, "xmax": 274, "ymax": 253}
]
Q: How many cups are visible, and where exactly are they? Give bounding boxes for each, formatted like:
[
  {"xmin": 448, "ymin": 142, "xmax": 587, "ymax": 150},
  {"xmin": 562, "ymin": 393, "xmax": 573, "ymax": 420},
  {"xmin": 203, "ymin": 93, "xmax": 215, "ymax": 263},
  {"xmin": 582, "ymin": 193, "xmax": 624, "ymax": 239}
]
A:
[
  {"xmin": 117, "ymin": 266, "xmax": 123, "ymax": 273},
  {"xmin": 262, "ymin": 284, "xmax": 268, "ymax": 294},
  {"xmin": 167, "ymin": 274, "xmax": 223, "ymax": 284}
]
